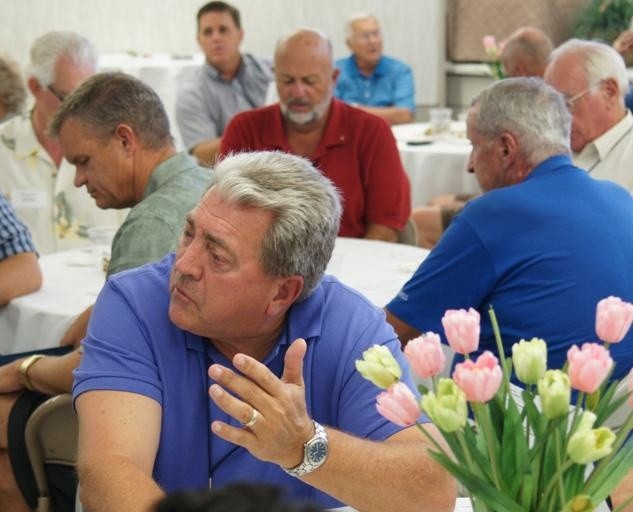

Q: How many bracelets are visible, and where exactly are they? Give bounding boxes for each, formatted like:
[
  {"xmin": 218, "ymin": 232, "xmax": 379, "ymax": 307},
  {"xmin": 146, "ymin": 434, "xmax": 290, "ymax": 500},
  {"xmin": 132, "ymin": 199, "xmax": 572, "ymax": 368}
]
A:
[{"xmin": 19, "ymin": 355, "xmax": 46, "ymax": 391}]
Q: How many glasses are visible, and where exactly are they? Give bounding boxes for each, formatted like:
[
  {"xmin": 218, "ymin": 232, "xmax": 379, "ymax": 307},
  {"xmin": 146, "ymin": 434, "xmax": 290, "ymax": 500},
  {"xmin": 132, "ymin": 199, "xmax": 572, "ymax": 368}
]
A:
[
  {"xmin": 47, "ymin": 85, "xmax": 66, "ymax": 103},
  {"xmin": 566, "ymin": 88, "xmax": 592, "ymax": 108}
]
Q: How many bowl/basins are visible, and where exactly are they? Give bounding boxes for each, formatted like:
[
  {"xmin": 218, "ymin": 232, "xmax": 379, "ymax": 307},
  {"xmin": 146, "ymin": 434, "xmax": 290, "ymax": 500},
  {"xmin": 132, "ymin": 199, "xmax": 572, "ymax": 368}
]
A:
[
  {"xmin": 70, "ymin": 267, "xmax": 106, "ymax": 295},
  {"xmin": 49, "ymin": 262, "xmax": 72, "ymax": 282}
]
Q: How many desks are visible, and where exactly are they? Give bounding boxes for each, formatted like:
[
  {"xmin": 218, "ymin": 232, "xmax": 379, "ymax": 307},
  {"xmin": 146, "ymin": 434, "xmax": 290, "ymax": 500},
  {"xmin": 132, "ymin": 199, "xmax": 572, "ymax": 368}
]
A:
[
  {"xmin": 389, "ymin": 119, "xmax": 510, "ymax": 203},
  {"xmin": 0, "ymin": 234, "xmax": 439, "ymax": 344}
]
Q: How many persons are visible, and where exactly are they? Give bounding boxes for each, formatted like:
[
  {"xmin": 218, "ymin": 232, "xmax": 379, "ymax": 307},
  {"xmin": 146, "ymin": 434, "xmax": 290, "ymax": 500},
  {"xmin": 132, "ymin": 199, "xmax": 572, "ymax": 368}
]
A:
[
  {"xmin": 613, "ymin": 28, "xmax": 633, "ymax": 109},
  {"xmin": 544, "ymin": 40, "xmax": 633, "ymax": 194},
  {"xmin": 0, "ymin": 196, "xmax": 43, "ymax": 307},
  {"xmin": 384, "ymin": 76, "xmax": 632, "ymax": 409},
  {"xmin": 502, "ymin": 26, "xmax": 553, "ymax": 78},
  {"xmin": 173, "ymin": 2, "xmax": 276, "ymax": 153},
  {"xmin": 73, "ymin": 152, "xmax": 458, "ymax": 512},
  {"xmin": 0, "ymin": 29, "xmax": 131, "ymax": 256},
  {"xmin": 0, "ymin": 71, "xmax": 215, "ymax": 512},
  {"xmin": 333, "ymin": 14, "xmax": 416, "ymax": 124},
  {"xmin": 217, "ymin": 28, "xmax": 410, "ymax": 243}
]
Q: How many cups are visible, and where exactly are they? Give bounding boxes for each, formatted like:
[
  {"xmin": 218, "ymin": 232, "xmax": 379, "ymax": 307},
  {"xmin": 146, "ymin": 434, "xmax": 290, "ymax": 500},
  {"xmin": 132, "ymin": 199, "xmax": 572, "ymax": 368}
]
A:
[
  {"xmin": 431, "ymin": 109, "xmax": 452, "ymax": 128},
  {"xmin": 89, "ymin": 226, "xmax": 118, "ymax": 266}
]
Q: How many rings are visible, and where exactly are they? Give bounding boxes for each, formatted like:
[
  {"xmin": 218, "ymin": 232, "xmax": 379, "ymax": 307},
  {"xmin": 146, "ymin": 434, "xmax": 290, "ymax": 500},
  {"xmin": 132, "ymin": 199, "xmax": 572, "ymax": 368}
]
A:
[{"xmin": 241, "ymin": 409, "xmax": 257, "ymax": 427}]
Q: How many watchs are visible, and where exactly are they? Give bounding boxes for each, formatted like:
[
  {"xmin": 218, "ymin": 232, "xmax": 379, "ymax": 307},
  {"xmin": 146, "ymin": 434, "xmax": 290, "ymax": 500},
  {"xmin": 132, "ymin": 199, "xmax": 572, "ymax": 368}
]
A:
[{"xmin": 281, "ymin": 419, "xmax": 330, "ymax": 477}]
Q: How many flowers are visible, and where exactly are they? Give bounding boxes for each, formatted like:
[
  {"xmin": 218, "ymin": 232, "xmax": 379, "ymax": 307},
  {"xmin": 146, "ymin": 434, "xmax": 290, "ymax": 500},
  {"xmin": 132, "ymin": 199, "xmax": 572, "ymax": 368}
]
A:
[
  {"xmin": 482, "ymin": 35, "xmax": 502, "ymax": 76},
  {"xmin": 354, "ymin": 294, "xmax": 632, "ymax": 512}
]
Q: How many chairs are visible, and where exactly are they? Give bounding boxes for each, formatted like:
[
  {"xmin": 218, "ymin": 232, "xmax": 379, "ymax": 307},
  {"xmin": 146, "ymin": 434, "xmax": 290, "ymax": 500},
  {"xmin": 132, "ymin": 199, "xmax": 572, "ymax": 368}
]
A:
[{"xmin": 25, "ymin": 392, "xmax": 81, "ymax": 512}]
